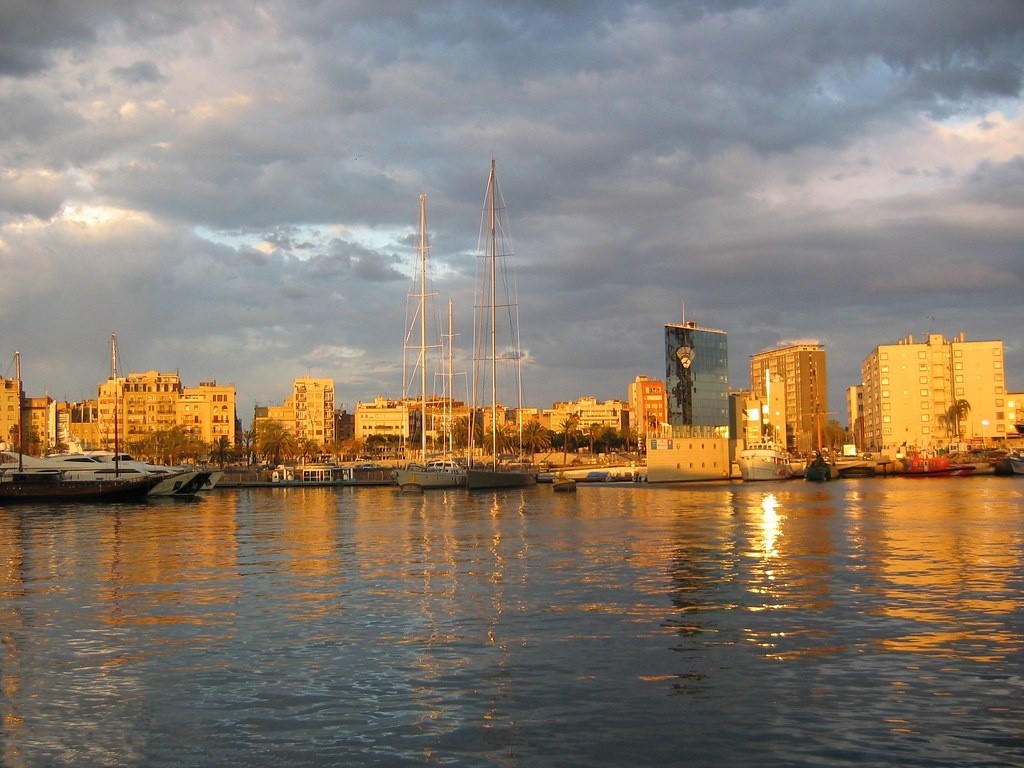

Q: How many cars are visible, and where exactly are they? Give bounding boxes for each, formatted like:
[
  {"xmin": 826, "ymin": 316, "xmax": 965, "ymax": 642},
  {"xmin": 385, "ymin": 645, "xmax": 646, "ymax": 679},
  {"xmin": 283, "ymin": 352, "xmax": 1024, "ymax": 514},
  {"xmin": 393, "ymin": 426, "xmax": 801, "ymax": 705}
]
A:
[
  {"xmin": 863, "ymin": 453, "xmax": 874, "ymax": 461},
  {"xmin": 363, "ymin": 463, "xmax": 375, "ymax": 468}
]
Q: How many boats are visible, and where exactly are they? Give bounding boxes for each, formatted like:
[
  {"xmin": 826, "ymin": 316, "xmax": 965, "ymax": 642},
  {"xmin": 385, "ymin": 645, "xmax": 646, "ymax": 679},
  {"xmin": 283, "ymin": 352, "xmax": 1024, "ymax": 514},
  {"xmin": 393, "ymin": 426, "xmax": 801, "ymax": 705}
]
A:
[
  {"xmin": 553, "ymin": 479, "xmax": 576, "ymax": 492},
  {"xmin": 0, "ymin": 450, "xmax": 225, "ymax": 496},
  {"xmin": 1010, "ymin": 456, "xmax": 1024, "ymax": 475},
  {"xmin": 737, "ymin": 428, "xmax": 793, "ymax": 481},
  {"xmin": 892, "ymin": 439, "xmax": 976, "ymax": 478},
  {"xmin": 837, "ymin": 466, "xmax": 876, "ymax": 478}
]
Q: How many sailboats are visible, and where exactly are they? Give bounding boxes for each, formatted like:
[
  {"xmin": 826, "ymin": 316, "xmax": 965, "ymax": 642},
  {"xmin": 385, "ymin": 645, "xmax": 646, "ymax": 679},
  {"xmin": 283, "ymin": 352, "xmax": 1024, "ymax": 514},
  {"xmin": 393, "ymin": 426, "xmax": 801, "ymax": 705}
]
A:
[
  {"xmin": 801, "ymin": 361, "xmax": 841, "ymax": 482},
  {"xmin": 0, "ymin": 332, "xmax": 189, "ymax": 504},
  {"xmin": 391, "ymin": 192, "xmax": 467, "ymax": 490},
  {"xmin": 466, "ymin": 158, "xmax": 539, "ymax": 491}
]
